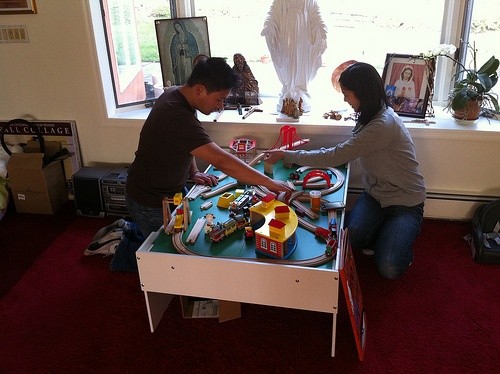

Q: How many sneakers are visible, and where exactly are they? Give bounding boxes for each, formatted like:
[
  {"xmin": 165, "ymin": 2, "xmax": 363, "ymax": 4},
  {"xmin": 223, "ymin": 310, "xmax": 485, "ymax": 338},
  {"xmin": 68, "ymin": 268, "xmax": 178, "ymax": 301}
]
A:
[
  {"xmin": 83, "ymin": 228, "xmax": 124, "ymax": 260},
  {"xmin": 90, "ymin": 219, "xmax": 129, "ymax": 243}
]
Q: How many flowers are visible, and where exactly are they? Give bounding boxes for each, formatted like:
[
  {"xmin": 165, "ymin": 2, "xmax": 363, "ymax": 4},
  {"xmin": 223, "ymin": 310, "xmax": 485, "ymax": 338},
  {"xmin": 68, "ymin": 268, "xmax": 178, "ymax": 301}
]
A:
[{"xmin": 425, "ymin": 39, "xmax": 500, "ymax": 113}]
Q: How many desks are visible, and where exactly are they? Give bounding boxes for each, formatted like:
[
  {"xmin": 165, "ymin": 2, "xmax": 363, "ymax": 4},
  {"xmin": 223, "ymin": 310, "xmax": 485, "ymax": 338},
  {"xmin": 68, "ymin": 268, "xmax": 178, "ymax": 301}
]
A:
[{"xmin": 134, "ymin": 147, "xmax": 351, "ymax": 357}]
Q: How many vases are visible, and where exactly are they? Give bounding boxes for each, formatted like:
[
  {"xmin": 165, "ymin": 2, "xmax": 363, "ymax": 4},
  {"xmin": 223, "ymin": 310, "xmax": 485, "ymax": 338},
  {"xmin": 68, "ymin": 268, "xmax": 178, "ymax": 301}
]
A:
[{"xmin": 453, "ymin": 97, "xmax": 479, "ymax": 119}]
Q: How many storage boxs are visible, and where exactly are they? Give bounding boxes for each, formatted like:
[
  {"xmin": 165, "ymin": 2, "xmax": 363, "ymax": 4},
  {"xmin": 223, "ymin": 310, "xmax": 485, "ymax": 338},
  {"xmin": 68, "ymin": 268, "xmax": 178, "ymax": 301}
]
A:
[{"xmin": 7, "ymin": 140, "xmax": 71, "ymax": 214}]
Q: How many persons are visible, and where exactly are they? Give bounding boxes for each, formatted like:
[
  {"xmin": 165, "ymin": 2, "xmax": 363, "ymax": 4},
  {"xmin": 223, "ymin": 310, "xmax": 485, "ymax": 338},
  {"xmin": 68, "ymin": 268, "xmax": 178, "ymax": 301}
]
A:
[
  {"xmin": 264, "ymin": 62, "xmax": 426, "ymax": 281},
  {"xmin": 125, "ymin": 57, "xmax": 293, "ymax": 236}
]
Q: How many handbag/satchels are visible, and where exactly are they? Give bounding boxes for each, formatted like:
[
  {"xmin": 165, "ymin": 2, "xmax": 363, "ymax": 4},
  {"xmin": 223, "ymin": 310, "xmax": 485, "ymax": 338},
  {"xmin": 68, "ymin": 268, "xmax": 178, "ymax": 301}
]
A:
[{"xmin": 464, "ymin": 201, "xmax": 500, "ymax": 264}]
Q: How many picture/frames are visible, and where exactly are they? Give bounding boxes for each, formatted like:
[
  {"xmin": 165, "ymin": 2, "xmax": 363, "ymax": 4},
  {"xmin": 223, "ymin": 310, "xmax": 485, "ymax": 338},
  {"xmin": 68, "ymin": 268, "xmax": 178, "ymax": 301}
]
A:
[
  {"xmin": 155, "ymin": 16, "xmax": 211, "ymax": 93},
  {"xmin": 0, "ymin": 0, "xmax": 37, "ymax": 15},
  {"xmin": 382, "ymin": 53, "xmax": 434, "ymax": 119}
]
